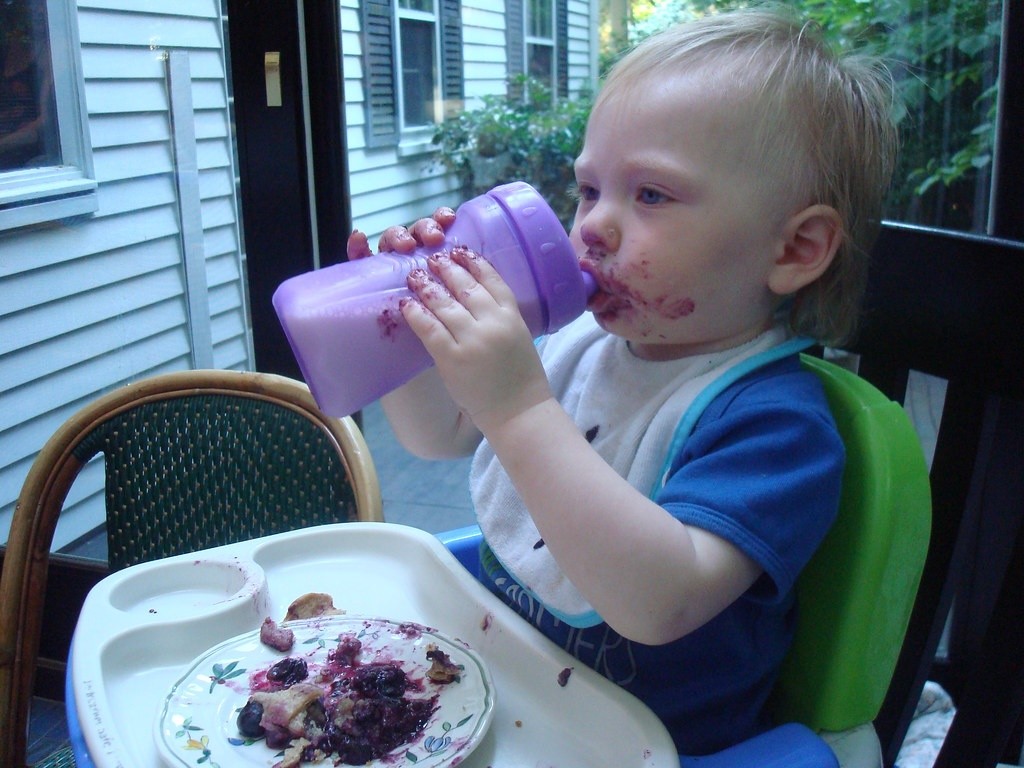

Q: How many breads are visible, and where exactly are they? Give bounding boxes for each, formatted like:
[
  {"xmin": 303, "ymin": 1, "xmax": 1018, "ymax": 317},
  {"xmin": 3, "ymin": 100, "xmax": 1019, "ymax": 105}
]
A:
[{"xmin": 283, "ymin": 591, "xmax": 346, "ymax": 625}]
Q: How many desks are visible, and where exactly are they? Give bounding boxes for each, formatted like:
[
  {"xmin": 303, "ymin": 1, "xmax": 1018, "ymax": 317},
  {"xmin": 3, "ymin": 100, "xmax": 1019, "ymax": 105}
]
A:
[{"xmin": 65, "ymin": 523, "xmax": 682, "ymax": 768}]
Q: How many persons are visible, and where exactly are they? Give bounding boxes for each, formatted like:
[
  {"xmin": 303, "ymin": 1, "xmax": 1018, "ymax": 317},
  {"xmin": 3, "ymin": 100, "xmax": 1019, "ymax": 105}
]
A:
[{"xmin": 346, "ymin": 14, "xmax": 901, "ymax": 757}]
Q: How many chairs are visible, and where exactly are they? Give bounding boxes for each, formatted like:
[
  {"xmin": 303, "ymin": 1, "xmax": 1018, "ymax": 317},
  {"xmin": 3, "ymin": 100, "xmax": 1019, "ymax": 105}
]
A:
[
  {"xmin": 0, "ymin": 369, "xmax": 383, "ymax": 768},
  {"xmin": 431, "ymin": 214, "xmax": 1024, "ymax": 767}
]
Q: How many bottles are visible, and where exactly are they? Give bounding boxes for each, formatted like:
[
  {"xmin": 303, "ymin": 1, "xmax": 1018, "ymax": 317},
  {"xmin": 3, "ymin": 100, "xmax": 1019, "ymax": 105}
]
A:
[{"xmin": 271, "ymin": 181, "xmax": 610, "ymax": 425}]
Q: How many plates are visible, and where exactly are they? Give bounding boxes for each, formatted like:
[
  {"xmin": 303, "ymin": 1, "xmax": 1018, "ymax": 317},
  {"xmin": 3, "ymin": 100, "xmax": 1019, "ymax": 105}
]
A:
[{"xmin": 154, "ymin": 615, "xmax": 494, "ymax": 768}]
